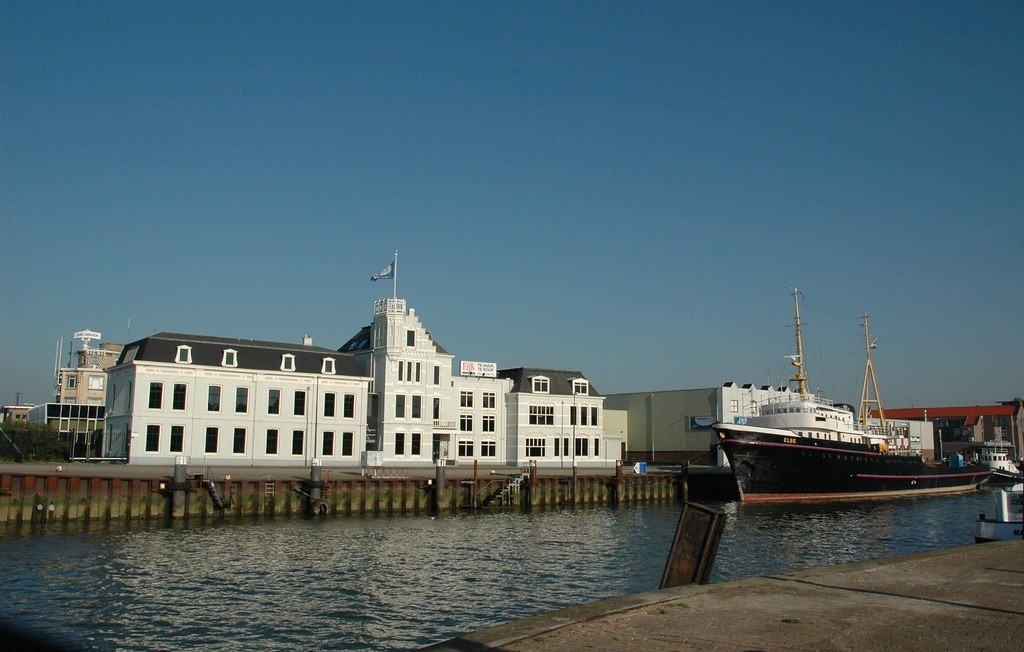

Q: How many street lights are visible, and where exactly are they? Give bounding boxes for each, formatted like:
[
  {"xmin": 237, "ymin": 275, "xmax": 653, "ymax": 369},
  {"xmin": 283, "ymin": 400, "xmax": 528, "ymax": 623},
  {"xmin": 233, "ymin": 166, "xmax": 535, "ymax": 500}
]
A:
[{"xmin": 572, "ymin": 391, "xmax": 578, "ymax": 466}]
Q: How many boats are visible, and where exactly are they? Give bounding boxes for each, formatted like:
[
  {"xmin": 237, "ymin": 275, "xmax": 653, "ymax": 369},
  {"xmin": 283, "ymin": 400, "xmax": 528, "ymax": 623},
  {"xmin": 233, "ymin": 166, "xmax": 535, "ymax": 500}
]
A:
[
  {"xmin": 712, "ymin": 287, "xmax": 995, "ymax": 505},
  {"xmin": 967, "ymin": 446, "xmax": 1024, "ymax": 483},
  {"xmin": 974, "ymin": 482, "xmax": 1024, "ymax": 544}
]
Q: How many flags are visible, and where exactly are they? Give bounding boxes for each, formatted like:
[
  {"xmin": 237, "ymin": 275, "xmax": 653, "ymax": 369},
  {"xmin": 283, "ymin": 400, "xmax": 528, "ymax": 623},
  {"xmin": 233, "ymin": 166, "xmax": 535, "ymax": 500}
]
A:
[
  {"xmin": 371, "ymin": 259, "xmax": 396, "ymax": 282},
  {"xmin": 864, "ymin": 337, "xmax": 878, "ymax": 349}
]
